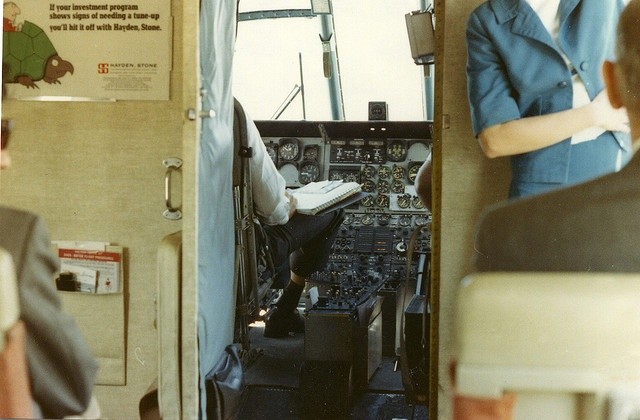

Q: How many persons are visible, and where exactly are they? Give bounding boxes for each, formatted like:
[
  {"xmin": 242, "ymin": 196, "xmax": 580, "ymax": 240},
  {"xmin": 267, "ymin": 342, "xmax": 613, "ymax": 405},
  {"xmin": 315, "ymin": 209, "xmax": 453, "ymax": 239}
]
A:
[
  {"xmin": 243, "ymin": 109, "xmax": 347, "ymax": 339},
  {"xmin": 465, "ymin": 0, "xmax": 634, "ymax": 207},
  {"xmin": 473, "ymin": 1, "xmax": 640, "ymax": 275},
  {"xmin": 1, "ymin": 61, "xmax": 103, "ymax": 420}
]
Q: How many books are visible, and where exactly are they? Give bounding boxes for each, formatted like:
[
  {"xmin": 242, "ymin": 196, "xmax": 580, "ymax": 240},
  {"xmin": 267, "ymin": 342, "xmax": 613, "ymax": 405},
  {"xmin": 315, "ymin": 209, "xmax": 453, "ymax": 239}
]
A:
[{"xmin": 285, "ymin": 180, "xmax": 372, "ymax": 216}]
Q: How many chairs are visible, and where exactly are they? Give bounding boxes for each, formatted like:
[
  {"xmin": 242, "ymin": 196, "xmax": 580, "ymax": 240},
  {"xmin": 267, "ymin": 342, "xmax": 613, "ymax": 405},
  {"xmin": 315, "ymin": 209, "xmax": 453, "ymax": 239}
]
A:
[
  {"xmin": 399, "ymin": 224, "xmax": 430, "ymax": 408},
  {"xmin": 231, "ymin": 98, "xmax": 286, "ymax": 369},
  {"xmin": 442, "ymin": 271, "xmax": 637, "ymax": 420},
  {"xmin": 0, "ymin": 250, "xmax": 101, "ymax": 420}
]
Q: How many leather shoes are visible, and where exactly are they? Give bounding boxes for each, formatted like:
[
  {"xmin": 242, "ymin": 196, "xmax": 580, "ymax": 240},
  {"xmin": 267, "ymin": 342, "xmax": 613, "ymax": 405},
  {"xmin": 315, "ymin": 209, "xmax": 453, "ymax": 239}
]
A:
[{"xmin": 265, "ymin": 309, "xmax": 306, "ymax": 332}]
